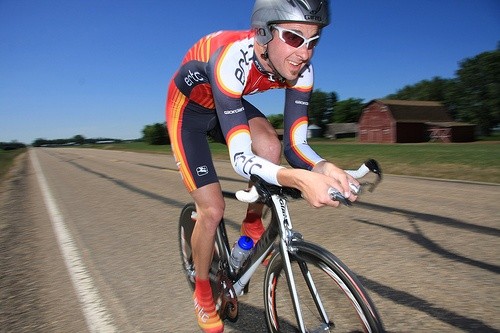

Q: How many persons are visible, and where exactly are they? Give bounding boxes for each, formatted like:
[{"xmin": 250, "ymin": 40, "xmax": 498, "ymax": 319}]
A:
[{"xmin": 165, "ymin": 0, "xmax": 361, "ymax": 332}]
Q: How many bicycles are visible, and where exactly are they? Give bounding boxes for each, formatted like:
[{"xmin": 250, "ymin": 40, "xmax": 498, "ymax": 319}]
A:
[{"xmin": 178, "ymin": 158, "xmax": 387, "ymax": 333}]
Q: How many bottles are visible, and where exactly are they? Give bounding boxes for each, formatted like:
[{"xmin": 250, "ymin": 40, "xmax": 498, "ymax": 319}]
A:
[{"xmin": 231, "ymin": 235, "xmax": 255, "ymax": 268}]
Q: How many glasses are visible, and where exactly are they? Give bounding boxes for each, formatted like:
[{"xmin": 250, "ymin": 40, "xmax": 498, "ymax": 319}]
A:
[{"xmin": 270, "ymin": 25, "xmax": 320, "ymax": 50}]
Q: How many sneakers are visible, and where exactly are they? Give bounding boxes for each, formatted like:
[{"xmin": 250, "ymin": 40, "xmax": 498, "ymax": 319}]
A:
[
  {"xmin": 191, "ymin": 277, "xmax": 225, "ymax": 333},
  {"xmin": 239, "ymin": 212, "xmax": 274, "ymax": 266}
]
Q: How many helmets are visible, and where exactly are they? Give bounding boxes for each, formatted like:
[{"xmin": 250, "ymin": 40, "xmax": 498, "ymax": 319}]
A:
[{"xmin": 250, "ymin": 0, "xmax": 330, "ymax": 46}]
